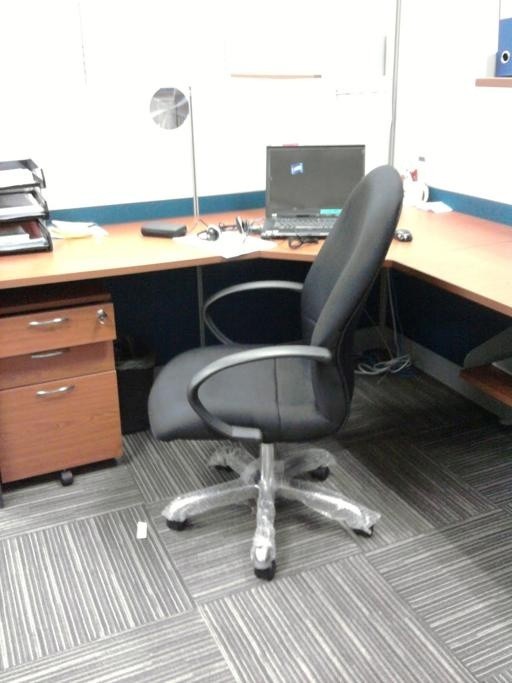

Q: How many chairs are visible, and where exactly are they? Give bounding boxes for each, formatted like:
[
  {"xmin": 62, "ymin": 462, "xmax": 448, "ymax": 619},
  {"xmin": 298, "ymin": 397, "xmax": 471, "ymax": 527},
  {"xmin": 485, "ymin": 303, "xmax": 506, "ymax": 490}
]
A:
[{"xmin": 146, "ymin": 162, "xmax": 405, "ymax": 581}]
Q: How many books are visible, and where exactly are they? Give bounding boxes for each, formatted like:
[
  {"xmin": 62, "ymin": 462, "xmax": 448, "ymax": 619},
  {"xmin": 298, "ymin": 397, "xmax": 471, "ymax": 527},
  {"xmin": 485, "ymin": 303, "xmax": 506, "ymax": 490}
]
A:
[{"xmin": 0, "ymin": 161, "xmax": 49, "ymax": 253}]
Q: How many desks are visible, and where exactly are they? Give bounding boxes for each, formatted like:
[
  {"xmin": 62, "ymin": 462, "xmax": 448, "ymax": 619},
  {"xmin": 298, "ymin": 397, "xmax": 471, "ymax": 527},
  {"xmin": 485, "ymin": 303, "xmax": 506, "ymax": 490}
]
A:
[{"xmin": 0, "ymin": 189, "xmax": 510, "ymax": 405}]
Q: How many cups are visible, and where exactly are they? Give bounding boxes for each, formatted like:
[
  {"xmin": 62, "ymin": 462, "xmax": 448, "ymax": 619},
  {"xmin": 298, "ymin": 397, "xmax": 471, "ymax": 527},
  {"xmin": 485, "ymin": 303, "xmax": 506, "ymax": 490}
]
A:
[{"xmin": 402, "ymin": 178, "xmax": 429, "ymax": 209}]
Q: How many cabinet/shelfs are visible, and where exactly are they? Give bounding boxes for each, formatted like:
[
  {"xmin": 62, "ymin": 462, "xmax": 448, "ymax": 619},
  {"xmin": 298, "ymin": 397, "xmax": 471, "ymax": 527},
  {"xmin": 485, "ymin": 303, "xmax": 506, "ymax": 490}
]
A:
[{"xmin": 0, "ymin": 289, "xmax": 121, "ymax": 487}]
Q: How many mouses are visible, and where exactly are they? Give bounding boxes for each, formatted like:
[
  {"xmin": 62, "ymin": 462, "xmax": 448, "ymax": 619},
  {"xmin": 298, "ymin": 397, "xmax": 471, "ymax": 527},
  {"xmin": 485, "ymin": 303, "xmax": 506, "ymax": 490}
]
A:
[{"xmin": 394, "ymin": 228, "xmax": 412, "ymax": 242}]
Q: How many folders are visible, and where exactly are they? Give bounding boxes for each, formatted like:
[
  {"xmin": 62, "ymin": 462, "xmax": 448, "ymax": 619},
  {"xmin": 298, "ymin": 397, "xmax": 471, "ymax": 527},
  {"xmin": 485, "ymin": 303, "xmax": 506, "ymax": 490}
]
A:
[{"xmin": 495, "ymin": 0, "xmax": 512, "ymax": 78}]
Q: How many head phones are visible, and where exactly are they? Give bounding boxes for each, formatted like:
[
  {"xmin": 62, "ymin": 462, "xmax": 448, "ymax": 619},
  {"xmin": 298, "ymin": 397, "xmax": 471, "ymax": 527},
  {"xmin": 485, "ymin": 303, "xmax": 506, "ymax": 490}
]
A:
[{"xmin": 196, "ymin": 214, "xmax": 248, "ymax": 241}]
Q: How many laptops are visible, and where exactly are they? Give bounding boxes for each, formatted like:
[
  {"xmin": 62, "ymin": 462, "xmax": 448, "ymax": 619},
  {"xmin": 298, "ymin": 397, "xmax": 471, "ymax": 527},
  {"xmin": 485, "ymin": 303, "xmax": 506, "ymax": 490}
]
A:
[{"xmin": 260, "ymin": 146, "xmax": 365, "ymax": 239}]
